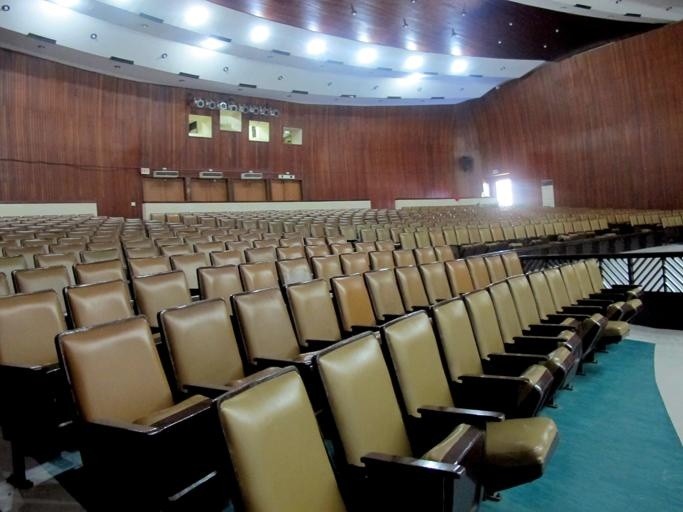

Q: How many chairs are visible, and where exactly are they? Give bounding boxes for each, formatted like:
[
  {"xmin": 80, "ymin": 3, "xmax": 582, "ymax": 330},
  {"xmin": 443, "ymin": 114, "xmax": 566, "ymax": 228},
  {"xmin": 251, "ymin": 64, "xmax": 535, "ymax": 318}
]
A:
[{"xmin": 0, "ymin": 204, "xmax": 683, "ymax": 512}]
